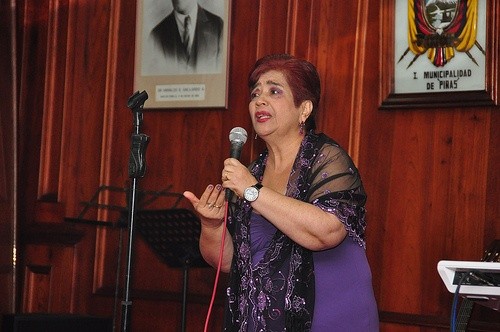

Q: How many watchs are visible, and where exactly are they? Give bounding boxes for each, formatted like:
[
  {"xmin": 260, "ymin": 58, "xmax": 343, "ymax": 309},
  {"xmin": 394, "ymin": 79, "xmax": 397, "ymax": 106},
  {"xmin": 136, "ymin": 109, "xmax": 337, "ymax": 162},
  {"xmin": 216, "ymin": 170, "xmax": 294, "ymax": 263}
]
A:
[{"xmin": 243, "ymin": 182, "xmax": 263, "ymax": 205}]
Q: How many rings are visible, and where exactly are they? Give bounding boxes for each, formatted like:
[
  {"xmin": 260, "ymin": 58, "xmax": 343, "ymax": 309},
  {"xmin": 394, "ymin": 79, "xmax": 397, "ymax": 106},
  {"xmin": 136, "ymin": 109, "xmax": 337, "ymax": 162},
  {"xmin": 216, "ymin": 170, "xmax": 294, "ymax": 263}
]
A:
[
  {"xmin": 207, "ymin": 203, "xmax": 213, "ymax": 206},
  {"xmin": 214, "ymin": 205, "xmax": 221, "ymax": 208}
]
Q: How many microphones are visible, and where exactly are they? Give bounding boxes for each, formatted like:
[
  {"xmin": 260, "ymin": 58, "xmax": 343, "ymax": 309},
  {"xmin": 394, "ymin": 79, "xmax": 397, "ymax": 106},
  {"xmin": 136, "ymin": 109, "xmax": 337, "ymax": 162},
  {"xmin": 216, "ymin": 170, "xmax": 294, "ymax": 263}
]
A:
[{"xmin": 225, "ymin": 126, "xmax": 248, "ymax": 202}]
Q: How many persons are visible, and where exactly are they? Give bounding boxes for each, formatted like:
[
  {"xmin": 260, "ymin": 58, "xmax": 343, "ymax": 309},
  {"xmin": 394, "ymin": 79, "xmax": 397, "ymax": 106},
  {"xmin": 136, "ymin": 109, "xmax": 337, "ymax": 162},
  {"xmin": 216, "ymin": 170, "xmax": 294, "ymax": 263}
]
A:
[{"xmin": 183, "ymin": 54, "xmax": 379, "ymax": 332}]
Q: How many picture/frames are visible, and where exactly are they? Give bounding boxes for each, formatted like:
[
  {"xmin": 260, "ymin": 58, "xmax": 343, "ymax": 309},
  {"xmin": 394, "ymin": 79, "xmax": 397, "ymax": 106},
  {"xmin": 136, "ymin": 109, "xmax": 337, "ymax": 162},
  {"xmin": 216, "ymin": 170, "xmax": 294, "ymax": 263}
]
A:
[
  {"xmin": 378, "ymin": 0, "xmax": 500, "ymax": 111},
  {"xmin": 133, "ymin": 0, "xmax": 232, "ymax": 109}
]
